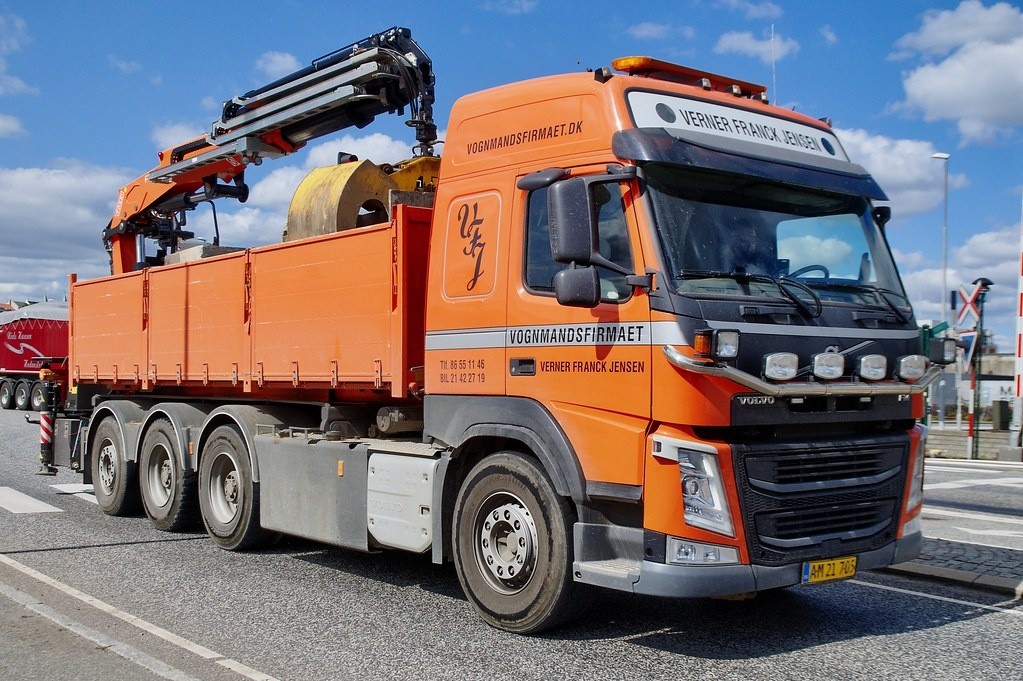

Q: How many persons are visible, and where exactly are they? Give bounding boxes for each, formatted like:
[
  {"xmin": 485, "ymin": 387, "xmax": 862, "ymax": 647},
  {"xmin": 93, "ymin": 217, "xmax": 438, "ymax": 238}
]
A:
[{"xmin": 725, "ymin": 219, "xmax": 778, "ymax": 275}]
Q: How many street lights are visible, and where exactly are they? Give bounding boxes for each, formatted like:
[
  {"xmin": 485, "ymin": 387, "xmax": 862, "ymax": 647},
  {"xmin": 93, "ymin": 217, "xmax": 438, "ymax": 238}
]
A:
[{"xmin": 931, "ymin": 152, "xmax": 950, "ymax": 431}]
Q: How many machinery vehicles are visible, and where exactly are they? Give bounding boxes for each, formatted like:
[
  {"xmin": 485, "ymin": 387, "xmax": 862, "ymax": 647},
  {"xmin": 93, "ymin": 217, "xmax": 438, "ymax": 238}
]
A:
[{"xmin": 101, "ymin": 24, "xmax": 446, "ymax": 276}]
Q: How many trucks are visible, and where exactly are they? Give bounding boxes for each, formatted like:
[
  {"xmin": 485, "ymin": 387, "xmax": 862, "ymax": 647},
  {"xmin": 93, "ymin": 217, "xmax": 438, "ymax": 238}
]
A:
[{"xmin": 0, "ymin": 301, "xmax": 69, "ymax": 412}]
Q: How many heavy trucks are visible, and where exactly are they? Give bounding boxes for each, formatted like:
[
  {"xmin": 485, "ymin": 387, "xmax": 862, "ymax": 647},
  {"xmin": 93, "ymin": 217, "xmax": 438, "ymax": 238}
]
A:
[{"xmin": 52, "ymin": 56, "xmax": 957, "ymax": 635}]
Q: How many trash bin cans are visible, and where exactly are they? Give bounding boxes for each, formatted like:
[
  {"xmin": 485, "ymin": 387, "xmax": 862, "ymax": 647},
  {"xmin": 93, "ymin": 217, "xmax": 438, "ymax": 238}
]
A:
[{"xmin": 992, "ymin": 400, "xmax": 1009, "ymax": 430}]
